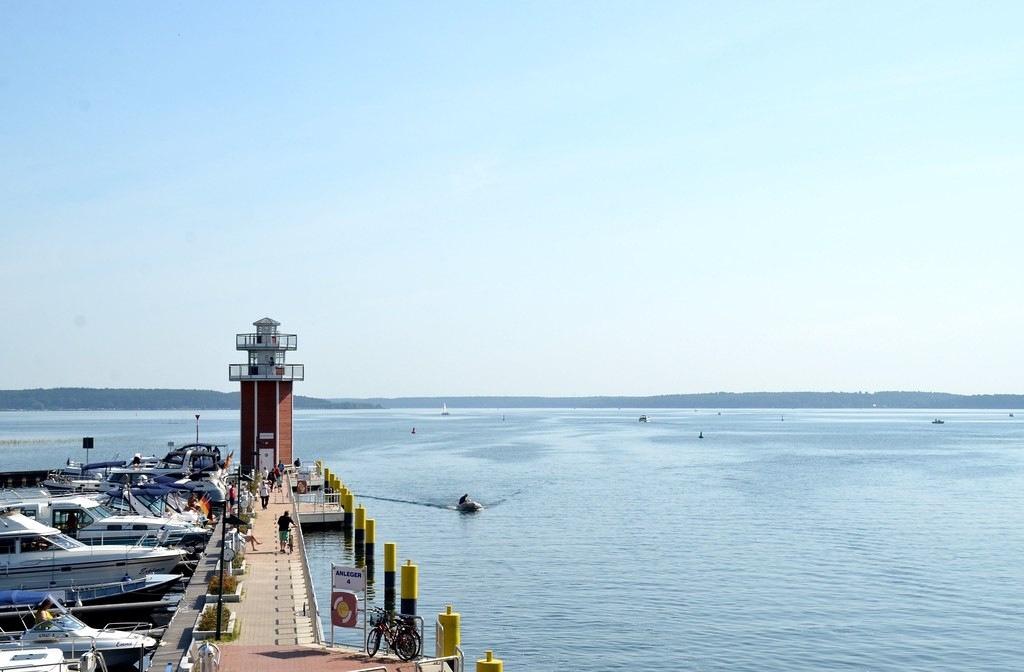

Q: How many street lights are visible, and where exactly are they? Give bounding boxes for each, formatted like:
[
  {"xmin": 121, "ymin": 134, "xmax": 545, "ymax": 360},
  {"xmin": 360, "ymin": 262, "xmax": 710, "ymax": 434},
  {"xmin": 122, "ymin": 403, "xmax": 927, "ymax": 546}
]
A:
[
  {"xmin": 249, "ymin": 447, "xmax": 261, "ymax": 493},
  {"xmin": 237, "ymin": 465, "xmax": 254, "ymax": 533},
  {"xmin": 216, "ymin": 501, "xmax": 248, "ymax": 640}
]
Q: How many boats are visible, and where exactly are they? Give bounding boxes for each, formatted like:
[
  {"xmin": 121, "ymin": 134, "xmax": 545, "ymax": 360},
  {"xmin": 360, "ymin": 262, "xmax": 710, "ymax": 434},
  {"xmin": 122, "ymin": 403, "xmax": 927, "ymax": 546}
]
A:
[
  {"xmin": 0, "ymin": 574, "xmax": 184, "ymax": 632},
  {"xmin": 0, "ymin": 508, "xmax": 195, "ymax": 591},
  {"xmin": 457, "ymin": 500, "xmax": 481, "ymax": 512},
  {"xmin": 0, "ymin": 443, "xmax": 227, "ymax": 546},
  {"xmin": 0, "ymin": 636, "xmax": 108, "ymax": 672},
  {"xmin": 0, "ymin": 593, "xmax": 156, "ymax": 670}
]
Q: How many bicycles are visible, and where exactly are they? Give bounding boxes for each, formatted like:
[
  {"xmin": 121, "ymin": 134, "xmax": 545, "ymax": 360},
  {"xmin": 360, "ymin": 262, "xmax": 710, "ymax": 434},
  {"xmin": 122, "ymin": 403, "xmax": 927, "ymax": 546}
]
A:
[
  {"xmin": 367, "ymin": 606, "xmax": 421, "ymax": 661},
  {"xmin": 287, "ymin": 526, "xmax": 296, "ymax": 554}
]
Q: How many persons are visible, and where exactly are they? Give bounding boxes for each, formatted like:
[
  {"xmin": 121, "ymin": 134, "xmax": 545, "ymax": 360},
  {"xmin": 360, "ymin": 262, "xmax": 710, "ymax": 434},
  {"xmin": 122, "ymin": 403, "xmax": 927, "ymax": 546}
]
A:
[
  {"xmin": 36, "ymin": 599, "xmax": 53, "ymax": 629},
  {"xmin": 263, "ymin": 460, "xmax": 285, "ymax": 492},
  {"xmin": 278, "ymin": 510, "xmax": 299, "ymax": 554},
  {"xmin": 213, "ymin": 446, "xmax": 220, "ymax": 460},
  {"xmin": 133, "ymin": 452, "xmax": 142, "ymax": 464},
  {"xmin": 231, "ymin": 523, "xmax": 263, "ymax": 551},
  {"xmin": 227, "ymin": 479, "xmax": 236, "ymax": 512},
  {"xmin": 187, "ymin": 494, "xmax": 201, "ymax": 511},
  {"xmin": 294, "ymin": 458, "xmax": 300, "ymax": 467},
  {"xmin": 267, "ymin": 357, "xmax": 275, "ymax": 375},
  {"xmin": 259, "ymin": 480, "xmax": 270, "ymax": 509},
  {"xmin": 459, "ymin": 493, "xmax": 468, "ymax": 504}
]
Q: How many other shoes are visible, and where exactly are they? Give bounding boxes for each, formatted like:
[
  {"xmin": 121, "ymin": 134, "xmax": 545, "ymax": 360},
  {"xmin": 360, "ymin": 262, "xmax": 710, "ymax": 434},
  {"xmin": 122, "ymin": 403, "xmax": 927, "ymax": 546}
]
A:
[
  {"xmin": 253, "ymin": 549, "xmax": 258, "ymax": 551},
  {"xmin": 257, "ymin": 542, "xmax": 262, "ymax": 544},
  {"xmin": 262, "ymin": 505, "xmax": 268, "ymax": 509},
  {"xmin": 280, "ymin": 549, "xmax": 286, "ymax": 553},
  {"xmin": 278, "ymin": 490, "xmax": 282, "ymax": 493}
]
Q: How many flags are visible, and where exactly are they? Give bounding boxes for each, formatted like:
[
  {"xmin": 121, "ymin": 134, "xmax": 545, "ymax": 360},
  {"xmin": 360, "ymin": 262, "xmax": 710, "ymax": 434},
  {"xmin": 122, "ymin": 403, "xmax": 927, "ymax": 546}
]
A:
[{"xmin": 199, "ymin": 492, "xmax": 213, "ymax": 520}]
